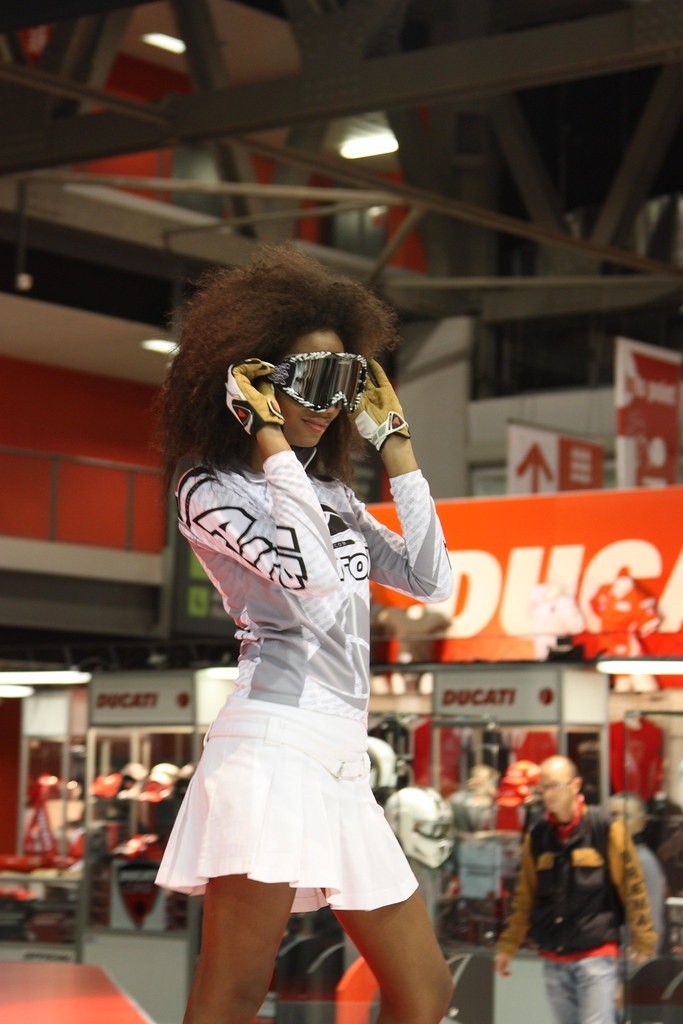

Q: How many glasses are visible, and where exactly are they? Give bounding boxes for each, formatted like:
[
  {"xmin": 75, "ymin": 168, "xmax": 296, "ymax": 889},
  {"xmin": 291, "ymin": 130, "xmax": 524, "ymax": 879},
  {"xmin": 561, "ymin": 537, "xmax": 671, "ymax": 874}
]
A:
[
  {"xmin": 610, "ymin": 810, "xmax": 635, "ymax": 821},
  {"xmin": 537, "ymin": 778, "xmax": 574, "ymax": 794},
  {"xmin": 260, "ymin": 351, "xmax": 369, "ymax": 414}
]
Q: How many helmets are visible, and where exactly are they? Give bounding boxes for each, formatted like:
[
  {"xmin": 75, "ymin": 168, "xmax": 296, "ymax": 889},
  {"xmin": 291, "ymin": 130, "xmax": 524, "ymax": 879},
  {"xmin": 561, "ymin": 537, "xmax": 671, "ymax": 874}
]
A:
[
  {"xmin": 368, "ymin": 736, "xmax": 398, "ymax": 790},
  {"xmin": 383, "ymin": 785, "xmax": 456, "ymax": 870}
]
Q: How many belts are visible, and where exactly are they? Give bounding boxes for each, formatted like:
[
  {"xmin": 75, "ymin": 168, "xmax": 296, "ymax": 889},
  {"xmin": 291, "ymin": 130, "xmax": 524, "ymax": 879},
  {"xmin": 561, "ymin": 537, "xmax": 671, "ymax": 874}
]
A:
[{"xmin": 206, "ymin": 720, "xmax": 371, "ymax": 778}]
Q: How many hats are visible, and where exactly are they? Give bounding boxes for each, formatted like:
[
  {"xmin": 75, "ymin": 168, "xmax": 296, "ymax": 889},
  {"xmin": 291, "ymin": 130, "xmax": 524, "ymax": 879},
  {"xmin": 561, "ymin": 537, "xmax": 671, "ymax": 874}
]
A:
[
  {"xmin": 92, "ymin": 763, "xmax": 194, "ymax": 801},
  {"xmin": 470, "ymin": 760, "xmax": 542, "ymax": 806}
]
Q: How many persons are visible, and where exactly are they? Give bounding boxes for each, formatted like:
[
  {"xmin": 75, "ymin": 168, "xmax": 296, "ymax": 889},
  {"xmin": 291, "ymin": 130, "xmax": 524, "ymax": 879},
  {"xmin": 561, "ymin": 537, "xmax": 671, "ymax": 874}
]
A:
[
  {"xmin": 493, "ymin": 755, "xmax": 664, "ymax": 1024},
  {"xmin": 155, "ymin": 248, "xmax": 455, "ymax": 1024}
]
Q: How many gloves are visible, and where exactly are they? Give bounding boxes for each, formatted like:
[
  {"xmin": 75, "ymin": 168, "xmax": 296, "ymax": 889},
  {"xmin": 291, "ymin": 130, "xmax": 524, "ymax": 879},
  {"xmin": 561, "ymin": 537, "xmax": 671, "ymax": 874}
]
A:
[
  {"xmin": 346, "ymin": 355, "xmax": 412, "ymax": 453},
  {"xmin": 224, "ymin": 357, "xmax": 287, "ymax": 440}
]
